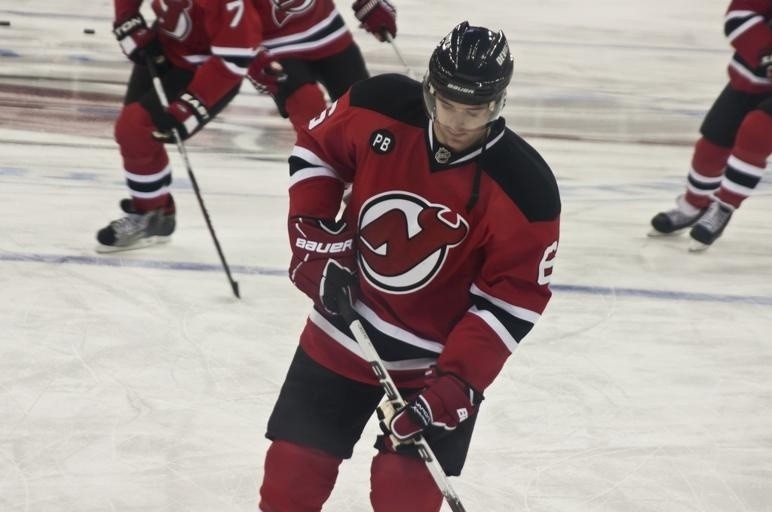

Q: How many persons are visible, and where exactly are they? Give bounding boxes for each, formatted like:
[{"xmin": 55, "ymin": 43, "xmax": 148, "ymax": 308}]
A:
[
  {"xmin": 259, "ymin": 21, "xmax": 562, "ymax": 512},
  {"xmin": 652, "ymin": 0, "xmax": 772, "ymax": 246},
  {"xmin": 94, "ymin": 0, "xmax": 264, "ymax": 249},
  {"xmin": 246, "ymin": 0, "xmax": 397, "ymax": 136}
]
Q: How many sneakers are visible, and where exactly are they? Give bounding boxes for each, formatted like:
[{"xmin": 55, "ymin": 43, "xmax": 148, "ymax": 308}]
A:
[
  {"xmin": 690, "ymin": 201, "xmax": 735, "ymax": 245},
  {"xmin": 651, "ymin": 194, "xmax": 707, "ymax": 233},
  {"xmin": 97, "ymin": 194, "xmax": 176, "ymax": 246}
]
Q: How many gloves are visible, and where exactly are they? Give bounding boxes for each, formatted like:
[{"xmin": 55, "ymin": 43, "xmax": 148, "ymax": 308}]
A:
[
  {"xmin": 114, "ymin": 14, "xmax": 161, "ymax": 64},
  {"xmin": 152, "ymin": 94, "xmax": 211, "ymax": 143},
  {"xmin": 352, "ymin": 0, "xmax": 397, "ymax": 43},
  {"xmin": 374, "ymin": 363, "xmax": 484, "ymax": 457},
  {"xmin": 287, "ymin": 217, "xmax": 359, "ymax": 314},
  {"xmin": 246, "ymin": 50, "xmax": 288, "ymax": 97}
]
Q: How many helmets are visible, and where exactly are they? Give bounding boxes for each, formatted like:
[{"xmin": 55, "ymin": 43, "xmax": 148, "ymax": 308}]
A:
[{"xmin": 422, "ymin": 21, "xmax": 514, "ymax": 131}]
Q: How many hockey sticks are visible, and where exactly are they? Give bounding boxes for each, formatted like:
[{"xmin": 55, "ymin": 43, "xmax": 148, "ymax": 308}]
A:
[{"xmin": 147, "ymin": 46, "xmax": 304, "ymax": 300}]
